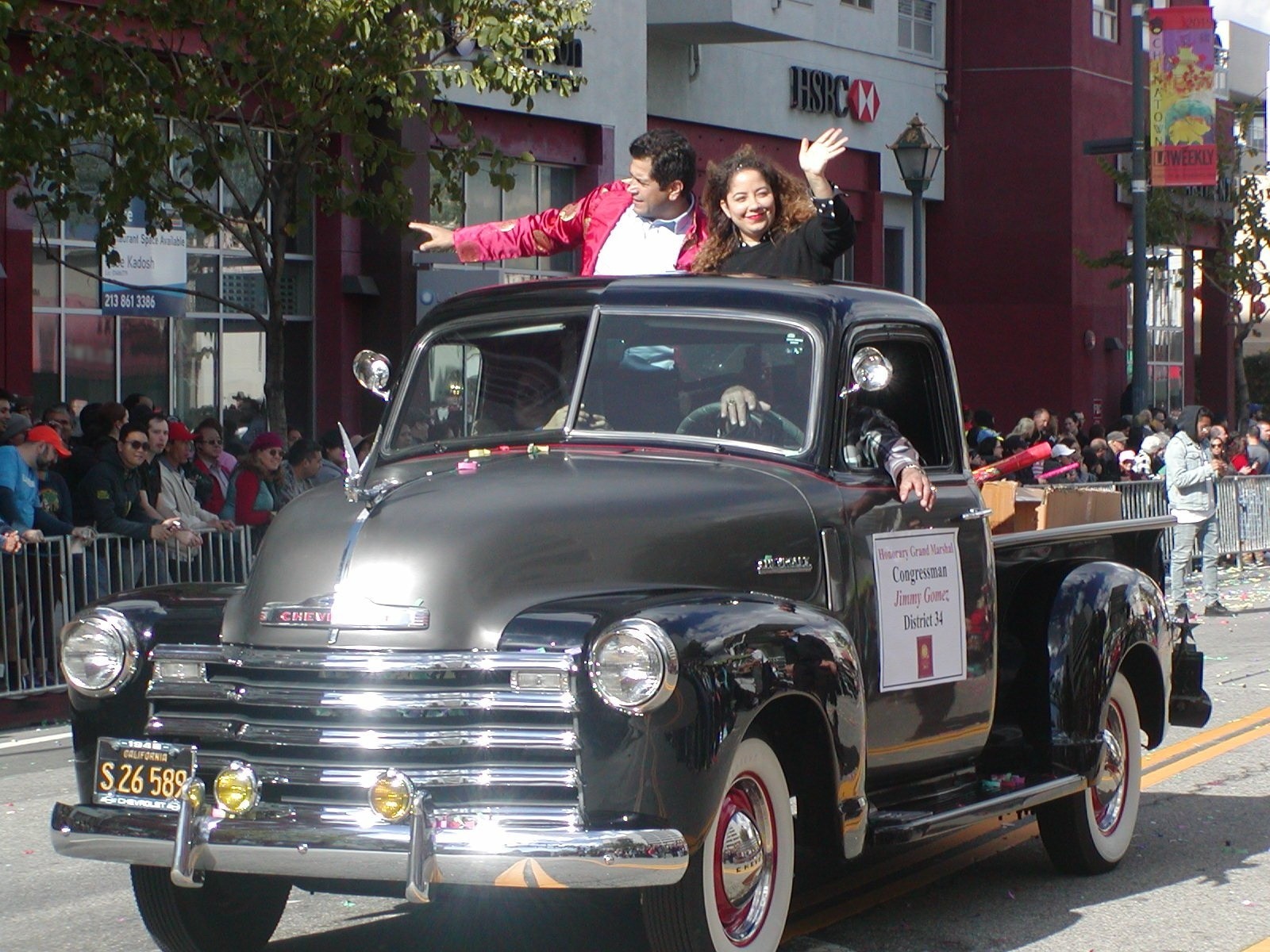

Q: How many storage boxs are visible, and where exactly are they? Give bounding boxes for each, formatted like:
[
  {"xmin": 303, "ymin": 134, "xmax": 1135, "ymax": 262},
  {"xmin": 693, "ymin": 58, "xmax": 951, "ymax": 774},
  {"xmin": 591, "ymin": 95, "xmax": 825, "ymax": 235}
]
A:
[{"xmin": 981, "ymin": 481, "xmax": 1122, "ymax": 535}]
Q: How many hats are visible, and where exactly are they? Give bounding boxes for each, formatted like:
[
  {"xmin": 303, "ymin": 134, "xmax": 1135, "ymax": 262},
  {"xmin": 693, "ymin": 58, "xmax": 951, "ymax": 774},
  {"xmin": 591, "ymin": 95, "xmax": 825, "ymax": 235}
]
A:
[
  {"xmin": 1120, "ymin": 450, "xmax": 1135, "ymax": 462},
  {"xmin": 1050, "ymin": 444, "xmax": 1075, "ymax": 458},
  {"xmin": 129, "ymin": 404, "xmax": 157, "ymax": 422},
  {"xmin": 977, "ymin": 429, "xmax": 1001, "ymax": 446},
  {"xmin": 26, "ymin": 426, "xmax": 72, "ymax": 457},
  {"xmin": 1108, "ymin": 431, "xmax": 1129, "ymax": 442},
  {"xmin": 1250, "ymin": 402, "xmax": 1263, "ymax": 413},
  {"xmin": 405, "ymin": 407, "xmax": 433, "ymax": 425},
  {"xmin": 967, "ymin": 426, "xmax": 1004, "ymax": 445},
  {"xmin": 350, "ymin": 432, "xmax": 377, "ymax": 450},
  {"xmin": 251, "ymin": 432, "xmax": 283, "ymax": 453},
  {"xmin": 168, "ymin": 421, "xmax": 201, "ymax": 441},
  {"xmin": 1044, "ymin": 434, "xmax": 1060, "ymax": 446},
  {"xmin": 2, "ymin": 414, "xmax": 31, "ymax": 442},
  {"xmin": 1004, "ymin": 436, "xmax": 1031, "ymax": 447}
]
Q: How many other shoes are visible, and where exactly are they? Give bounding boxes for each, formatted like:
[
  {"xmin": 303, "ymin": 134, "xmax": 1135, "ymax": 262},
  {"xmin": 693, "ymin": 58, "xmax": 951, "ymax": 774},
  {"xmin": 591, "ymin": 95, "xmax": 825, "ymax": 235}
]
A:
[
  {"xmin": 23, "ymin": 671, "xmax": 61, "ymax": 686},
  {"xmin": 1189, "ymin": 556, "xmax": 1265, "ymax": 576}
]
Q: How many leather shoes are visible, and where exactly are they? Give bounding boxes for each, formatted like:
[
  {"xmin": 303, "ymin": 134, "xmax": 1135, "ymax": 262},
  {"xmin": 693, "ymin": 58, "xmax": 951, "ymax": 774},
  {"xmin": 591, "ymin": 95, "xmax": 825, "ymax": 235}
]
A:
[
  {"xmin": 1175, "ymin": 603, "xmax": 1198, "ymax": 619},
  {"xmin": 1205, "ymin": 602, "xmax": 1238, "ymax": 616}
]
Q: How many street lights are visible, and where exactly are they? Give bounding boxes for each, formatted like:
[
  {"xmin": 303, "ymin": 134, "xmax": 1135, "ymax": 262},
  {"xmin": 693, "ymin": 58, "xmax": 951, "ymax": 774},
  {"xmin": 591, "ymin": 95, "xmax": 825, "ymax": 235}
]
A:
[{"xmin": 883, "ymin": 110, "xmax": 951, "ymax": 305}]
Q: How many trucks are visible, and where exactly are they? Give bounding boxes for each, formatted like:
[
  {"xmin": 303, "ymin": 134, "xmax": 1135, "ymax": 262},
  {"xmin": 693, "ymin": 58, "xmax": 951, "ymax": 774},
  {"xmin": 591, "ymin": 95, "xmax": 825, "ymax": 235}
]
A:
[{"xmin": 49, "ymin": 271, "xmax": 1232, "ymax": 951}]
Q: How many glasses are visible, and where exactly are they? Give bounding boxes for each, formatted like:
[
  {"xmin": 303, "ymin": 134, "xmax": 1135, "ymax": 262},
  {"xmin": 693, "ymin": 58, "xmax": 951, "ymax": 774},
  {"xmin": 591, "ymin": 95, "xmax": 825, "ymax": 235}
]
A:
[
  {"xmin": 55, "ymin": 419, "xmax": 72, "ymax": 430},
  {"xmin": 263, "ymin": 449, "xmax": 287, "ymax": 458},
  {"xmin": 1154, "ymin": 416, "xmax": 1166, "ymax": 423},
  {"xmin": 124, "ymin": 439, "xmax": 151, "ymax": 452},
  {"xmin": 198, "ymin": 439, "xmax": 223, "ymax": 445},
  {"xmin": 1211, "ymin": 443, "xmax": 1222, "ymax": 448},
  {"xmin": 1097, "ymin": 447, "xmax": 1108, "ymax": 451},
  {"xmin": 1197, "ymin": 426, "xmax": 1212, "ymax": 432}
]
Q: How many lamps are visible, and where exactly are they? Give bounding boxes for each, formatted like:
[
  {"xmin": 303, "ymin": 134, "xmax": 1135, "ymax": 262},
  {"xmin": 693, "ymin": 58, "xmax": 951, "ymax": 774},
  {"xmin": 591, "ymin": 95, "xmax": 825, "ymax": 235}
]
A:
[
  {"xmin": 345, "ymin": 275, "xmax": 377, "ymax": 294},
  {"xmin": 1105, "ymin": 337, "xmax": 1123, "ymax": 350}
]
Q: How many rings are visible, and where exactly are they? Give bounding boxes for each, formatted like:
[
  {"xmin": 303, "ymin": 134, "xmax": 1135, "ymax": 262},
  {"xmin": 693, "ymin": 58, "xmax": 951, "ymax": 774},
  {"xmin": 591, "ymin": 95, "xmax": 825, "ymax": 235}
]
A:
[
  {"xmin": 931, "ymin": 486, "xmax": 937, "ymax": 493},
  {"xmin": 729, "ymin": 400, "xmax": 735, "ymax": 405}
]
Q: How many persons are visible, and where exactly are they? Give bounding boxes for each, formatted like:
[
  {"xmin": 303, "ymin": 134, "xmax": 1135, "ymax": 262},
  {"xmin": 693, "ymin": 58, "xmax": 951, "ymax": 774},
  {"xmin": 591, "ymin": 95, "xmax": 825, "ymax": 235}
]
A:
[
  {"xmin": 0, "ymin": 388, "xmax": 375, "ymax": 701},
  {"xmin": 689, "ymin": 127, "xmax": 858, "ymax": 382},
  {"xmin": 964, "ymin": 404, "xmax": 1129, "ymax": 484},
  {"xmin": 1120, "ymin": 404, "xmax": 1270, "ymax": 575},
  {"xmin": 409, "ymin": 128, "xmax": 708, "ymax": 379},
  {"xmin": 397, "ymin": 375, "xmax": 478, "ymax": 446},
  {"xmin": 511, "ymin": 363, "xmax": 615, "ymax": 431},
  {"xmin": 1163, "ymin": 405, "xmax": 1238, "ymax": 618},
  {"xmin": 719, "ymin": 345, "xmax": 936, "ymax": 514}
]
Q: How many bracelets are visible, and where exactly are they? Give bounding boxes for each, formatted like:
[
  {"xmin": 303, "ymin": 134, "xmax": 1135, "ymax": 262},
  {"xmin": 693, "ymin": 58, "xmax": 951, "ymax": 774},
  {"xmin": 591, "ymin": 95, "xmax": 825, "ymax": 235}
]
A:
[{"xmin": 906, "ymin": 464, "xmax": 925, "ymax": 473}]
